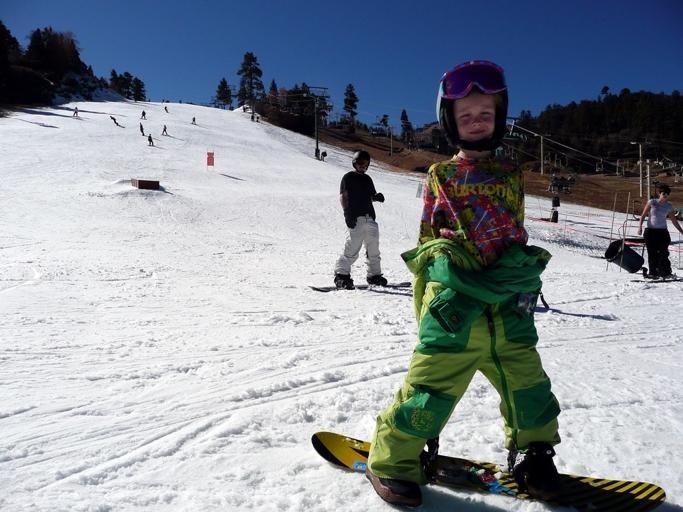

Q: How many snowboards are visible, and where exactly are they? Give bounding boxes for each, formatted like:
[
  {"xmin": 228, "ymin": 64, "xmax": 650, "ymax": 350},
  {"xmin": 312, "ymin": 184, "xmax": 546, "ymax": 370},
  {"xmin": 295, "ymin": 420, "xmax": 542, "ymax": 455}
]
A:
[
  {"xmin": 630, "ymin": 277, "xmax": 683, "ymax": 282},
  {"xmin": 311, "ymin": 432, "xmax": 667, "ymax": 512},
  {"xmin": 309, "ymin": 281, "xmax": 410, "ymax": 292}
]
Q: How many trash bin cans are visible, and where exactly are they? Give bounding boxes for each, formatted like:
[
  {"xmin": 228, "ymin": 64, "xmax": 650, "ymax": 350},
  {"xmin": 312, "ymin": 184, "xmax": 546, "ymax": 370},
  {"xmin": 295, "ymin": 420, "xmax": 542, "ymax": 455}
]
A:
[{"xmin": 605, "ymin": 240, "xmax": 644, "ymax": 273}]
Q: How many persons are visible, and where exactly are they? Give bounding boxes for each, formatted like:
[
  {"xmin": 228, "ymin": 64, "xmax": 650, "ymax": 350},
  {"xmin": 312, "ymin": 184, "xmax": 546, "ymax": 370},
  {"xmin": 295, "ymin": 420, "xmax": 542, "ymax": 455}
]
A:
[
  {"xmin": 638, "ymin": 184, "xmax": 682, "ymax": 278},
  {"xmin": 191, "ymin": 116, "xmax": 196, "ymax": 124},
  {"xmin": 139, "ymin": 110, "xmax": 145, "ymax": 120},
  {"xmin": 71, "ymin": 106, "xmax": 78, "ymax": 117},
  {"xmin": 565, "ymin": 175, "xmax": 575, "ymax": 194},
  {"xmin": 319, "ymin": 150, "xmax": 326, "ymax": 161},
  {"xmin": 164, "ymin": 107, "xmax": 168, "ymax": 114},
  {"xmin": 365, "ymin": 59, "xmax": 563, "ymax": 508},
  {"xmin": 160, "ymin": 124, "xmax": 167, "ymax": 136},
  {"xmin": 332, "ymin": 150, "xmax": 387, "ymax": 290},
  {"xmin": 147, "ymin": 134, "xmax": 152, "ymax": 147},
  {"xmin": 109, "ymin": 116, "xmax": 120, "ymax": 127},
  {"xmin": 139, "ymin": 123, "xmax": 144, "ymax": 136},
  {"xmin": 544, "ymin": 173, "xmax": 556, "ymax": 192}
]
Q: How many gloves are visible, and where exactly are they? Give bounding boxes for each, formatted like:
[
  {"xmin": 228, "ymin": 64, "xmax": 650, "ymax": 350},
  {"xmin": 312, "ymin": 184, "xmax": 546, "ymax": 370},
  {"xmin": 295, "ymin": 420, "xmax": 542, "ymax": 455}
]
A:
[
  {"xmin": 375, "ymin": 192, "xmax": 385, "ymax": 203},
  {"xmin": 343, "ymin": 208, "xmax": 357, "ymax": 229}
]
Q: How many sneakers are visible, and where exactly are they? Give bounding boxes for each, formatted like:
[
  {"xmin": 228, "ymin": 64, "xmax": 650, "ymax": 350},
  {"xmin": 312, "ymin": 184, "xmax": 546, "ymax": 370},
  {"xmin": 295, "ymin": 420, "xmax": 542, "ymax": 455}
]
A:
[
  {"xmin": 364, "ymin": 465, "xmax": 424, "ymax": 510},
  {"xmin": 514, "ymin": 460, "xmax": 567, "ymax": 506},
  {"xmin": 334, "ymin": 274, "xmax": 356, "ymax": 290},
  {"xmin": 366, "ymin": 275, "xmax": 387, "ymax": 287}
]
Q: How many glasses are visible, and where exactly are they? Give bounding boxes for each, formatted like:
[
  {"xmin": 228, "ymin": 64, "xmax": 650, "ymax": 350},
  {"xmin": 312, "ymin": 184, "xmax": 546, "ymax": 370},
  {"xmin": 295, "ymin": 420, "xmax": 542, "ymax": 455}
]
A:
[
  {"xmin": 438, "ymin": 59, "xmax": 508, "ymax": 100},
  {"xmin": 661, "ymin": 192, "xmax": 669, "ymax": 196}
]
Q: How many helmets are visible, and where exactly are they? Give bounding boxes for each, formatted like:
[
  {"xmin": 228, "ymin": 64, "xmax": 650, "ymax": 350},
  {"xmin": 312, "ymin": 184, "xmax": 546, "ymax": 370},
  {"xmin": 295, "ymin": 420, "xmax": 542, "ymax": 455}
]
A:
[
  {"xmin": 351, "ymin": 150, "xmax": 370, "ymax": 173},
  {"xmin": 433, "ymin": 58, "xmax": 511, "ymax": 153}
]
